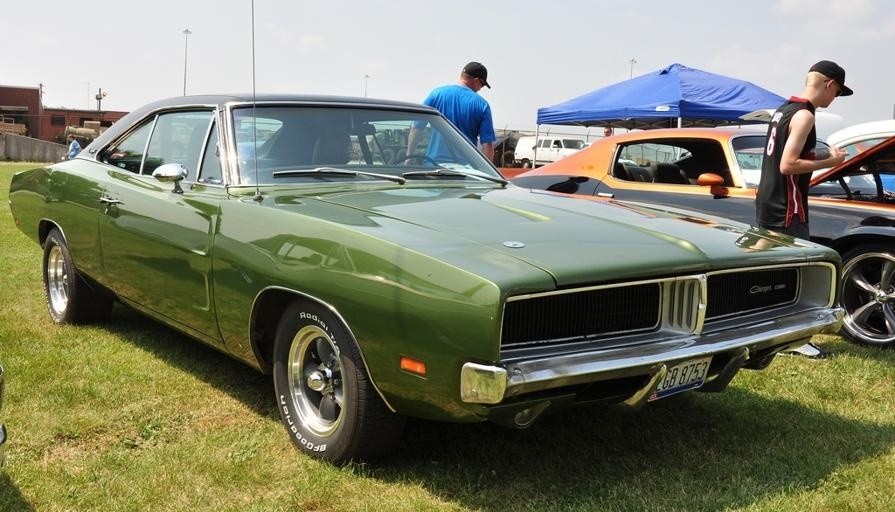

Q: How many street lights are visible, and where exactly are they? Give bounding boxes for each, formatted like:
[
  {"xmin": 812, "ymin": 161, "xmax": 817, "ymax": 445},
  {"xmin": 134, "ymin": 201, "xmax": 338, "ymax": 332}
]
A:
[
  {"xmin": 630, "ymin": 58, "xmax": 636, "ymax": 77},
  {"xmin": 183, "ymin": 24, "xmax": 191, "ymax": 96},
  {"xmin": 365, "ymin": 73, "xmax": 370, "ymax": 97}
]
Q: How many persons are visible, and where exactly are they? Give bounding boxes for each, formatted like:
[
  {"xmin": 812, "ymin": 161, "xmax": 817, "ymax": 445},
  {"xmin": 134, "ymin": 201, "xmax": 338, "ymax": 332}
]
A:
[
  {"xmin": 61, "ymin": 134, "xmax": 83, "ymax": 161},
  {"xmin": 755, "ymin": 60, "xmax": 853, "ymax": 358},
  {"xmin": 405, "ymin": 62, "xmax": 497, "ymax": 167}
]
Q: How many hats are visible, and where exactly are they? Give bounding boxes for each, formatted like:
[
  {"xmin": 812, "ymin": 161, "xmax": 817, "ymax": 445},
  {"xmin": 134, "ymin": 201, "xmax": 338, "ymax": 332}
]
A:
[
  {"xmin": 808, "ymin": 60, "xmax": 854, "ymax": 96},
  {"xmin": 464, "ymin": 64, "xmax": 490, "ymax": 88}
]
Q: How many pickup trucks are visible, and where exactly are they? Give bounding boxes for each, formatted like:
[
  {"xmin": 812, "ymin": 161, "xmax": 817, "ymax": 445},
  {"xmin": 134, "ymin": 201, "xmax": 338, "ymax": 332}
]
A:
[{"xmin": 0, "ymin": 114, "xmax": 27, "ymax": 135}]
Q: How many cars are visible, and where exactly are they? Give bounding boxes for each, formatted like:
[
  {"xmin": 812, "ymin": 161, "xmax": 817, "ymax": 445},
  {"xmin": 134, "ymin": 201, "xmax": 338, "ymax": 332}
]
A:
[
  {"xmin": 508, "ymin": 128, "xmax": 883, "ymax": 345},
  {"xmin": 10, "ymin": 94, "xmax": 846, "ymax": 466},
  {"xmin": 737, "ymin": 109, "xmax": 895, "ymax": 194}
]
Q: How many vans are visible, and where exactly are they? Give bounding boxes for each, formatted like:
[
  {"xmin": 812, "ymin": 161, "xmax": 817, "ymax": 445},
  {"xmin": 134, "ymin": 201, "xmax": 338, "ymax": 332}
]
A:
[{"xmin": 514, "ymin": 136, "xmax": 589, "ymax": 168}]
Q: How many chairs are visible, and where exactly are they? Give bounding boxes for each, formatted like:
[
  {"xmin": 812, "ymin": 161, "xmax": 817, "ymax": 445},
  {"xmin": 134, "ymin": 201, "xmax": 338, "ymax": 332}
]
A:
[
  {"xmin": 644, "ymin": 162, "xmax": 689, "ymax": 184},
  {"xmin": 623, "ymin": 166, "xmax": 652, "ymax": 182},
  {"xmin": 311, "ymin": 132, "xmax": 355, "ymax": 165}
]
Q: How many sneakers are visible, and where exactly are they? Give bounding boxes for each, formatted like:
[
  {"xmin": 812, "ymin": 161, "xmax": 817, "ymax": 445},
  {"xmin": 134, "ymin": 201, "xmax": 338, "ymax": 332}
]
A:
[{"xmin": 777, "ymin": 342, "xmax": 828, "ymax": 361}]
